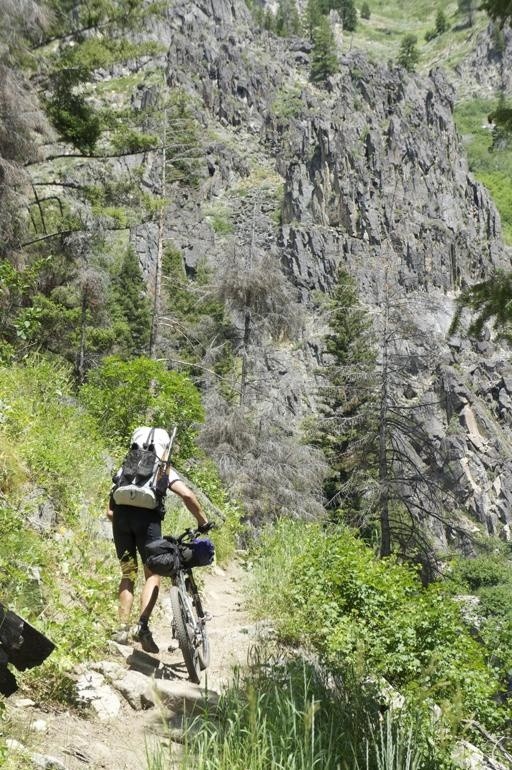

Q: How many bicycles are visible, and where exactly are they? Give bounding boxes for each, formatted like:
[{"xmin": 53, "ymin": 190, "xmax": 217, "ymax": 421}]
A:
[{"xmin": 163, "ymin": 521, "xmax": 215, "ymax": 684}]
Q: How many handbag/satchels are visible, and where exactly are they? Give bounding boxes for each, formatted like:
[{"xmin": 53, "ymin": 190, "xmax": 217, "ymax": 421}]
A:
[
  {"xmin": 144, "ymin": 534, "xmax": 176, "ymax": 557},
  {"xmin": 147, "ymin": 551, "xmax": 179, "ymax": 577},
  {"xmin": 185, "ymin": 536, "xmax": 215, "ymax": 567}
]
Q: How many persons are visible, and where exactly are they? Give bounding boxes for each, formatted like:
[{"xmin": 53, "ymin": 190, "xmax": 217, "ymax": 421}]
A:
[{"xmin": 107, "ymin": 462, "xmax": 209, "ymax": 655}]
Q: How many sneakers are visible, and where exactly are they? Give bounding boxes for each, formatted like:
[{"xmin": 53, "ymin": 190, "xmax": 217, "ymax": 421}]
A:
[
  {"xmin": 133, "ymin": 625, "xmax": 160, "ymax": 655},
  {"xmin": 113, "ymin": 631, "xmax": 130, "ymax": 645}
]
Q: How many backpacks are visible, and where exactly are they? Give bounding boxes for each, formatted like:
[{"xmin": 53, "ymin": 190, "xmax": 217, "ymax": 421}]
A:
[{"xmin": 109, "ymin": 424, "xmax": 177, "ymax": 512}]
